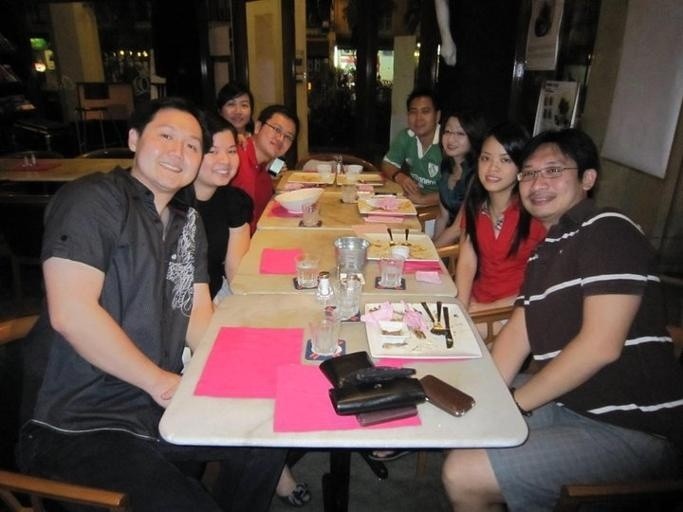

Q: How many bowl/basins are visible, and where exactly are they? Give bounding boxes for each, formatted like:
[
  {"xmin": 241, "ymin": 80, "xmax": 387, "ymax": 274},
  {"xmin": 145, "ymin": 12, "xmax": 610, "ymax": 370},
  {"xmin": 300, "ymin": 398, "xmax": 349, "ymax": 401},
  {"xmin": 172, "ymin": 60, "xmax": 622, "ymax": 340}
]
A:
[
  {"xmin": 271, "ymin": 188, "xmax": 324, "ymax": 214},
  {"xmin": 348, "ymin": 165, "xmax": 363, "ymax": 173}
]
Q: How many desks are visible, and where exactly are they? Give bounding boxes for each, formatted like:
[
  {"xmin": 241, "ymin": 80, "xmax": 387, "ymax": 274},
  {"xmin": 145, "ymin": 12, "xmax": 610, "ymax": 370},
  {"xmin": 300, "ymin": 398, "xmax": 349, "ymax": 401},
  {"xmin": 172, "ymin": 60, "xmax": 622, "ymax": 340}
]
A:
[{"xmin": 0, "ymin": 157, "xmax": 138, "ymax": 308}]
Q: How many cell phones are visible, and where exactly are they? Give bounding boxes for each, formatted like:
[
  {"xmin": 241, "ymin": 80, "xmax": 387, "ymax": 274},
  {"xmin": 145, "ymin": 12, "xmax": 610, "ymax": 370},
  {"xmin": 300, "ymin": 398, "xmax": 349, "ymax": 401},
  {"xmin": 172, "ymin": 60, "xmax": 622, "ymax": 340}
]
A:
[
  {"xmin": 418, "ymin": 375, "xmax": 476, "ymax": 416},
  {"xmin": 268, "ymin": 156, "xmax": 287, "ymax": 176}
]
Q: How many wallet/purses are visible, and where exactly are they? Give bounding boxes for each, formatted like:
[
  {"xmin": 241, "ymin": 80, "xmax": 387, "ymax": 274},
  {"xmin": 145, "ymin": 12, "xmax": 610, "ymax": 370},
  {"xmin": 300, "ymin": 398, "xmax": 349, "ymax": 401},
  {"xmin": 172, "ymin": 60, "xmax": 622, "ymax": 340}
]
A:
[
  {"xmin": 319, "ymin": 351, "xmax": 374, "ymax": 388},
  {"xmin": 328, "ymin": 378, "xmax": 427, "ymax": 416},
  {"xmin": 357, "ymin": 406, "xmax": 419, "ymax": 427},
  {"xmin": 348, "ymin": 365, "xmax": 417, "ymax": 387}
]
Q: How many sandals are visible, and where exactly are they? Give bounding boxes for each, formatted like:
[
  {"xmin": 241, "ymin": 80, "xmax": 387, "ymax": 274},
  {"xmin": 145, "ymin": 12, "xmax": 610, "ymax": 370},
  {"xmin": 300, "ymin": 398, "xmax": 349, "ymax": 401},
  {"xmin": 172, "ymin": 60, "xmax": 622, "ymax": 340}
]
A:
[{"xmin": 369, "ymin": 450, "xmax": 413, "ymax": 461}]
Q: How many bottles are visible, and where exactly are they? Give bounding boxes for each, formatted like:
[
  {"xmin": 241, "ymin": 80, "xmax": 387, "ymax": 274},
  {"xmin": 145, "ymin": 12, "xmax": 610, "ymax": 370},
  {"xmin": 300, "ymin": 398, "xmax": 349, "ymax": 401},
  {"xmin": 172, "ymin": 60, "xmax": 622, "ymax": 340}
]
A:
[
  {"xmin": 336, "ymin": 160, "xmax": 341, "ymax": 173},
  {"xmin": 316, "ymin": 271, "xmax": 333, "ymax": 306}
]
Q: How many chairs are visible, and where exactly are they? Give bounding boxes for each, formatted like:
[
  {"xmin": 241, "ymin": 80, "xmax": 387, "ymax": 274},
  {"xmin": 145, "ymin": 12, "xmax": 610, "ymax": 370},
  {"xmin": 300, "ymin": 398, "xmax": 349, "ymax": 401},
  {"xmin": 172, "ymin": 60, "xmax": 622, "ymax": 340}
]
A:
[
  {"xmin": 549, "ymin": 326, "xmax": 682, "ymax": 512},
  {"xmin": 77, "ymin": 147, "xmax": 134, "ymax": 160},
  {"xmin": 7, "ymin": 150, "xmax": 68, "ymax": 159},
  {"xmin": 0, "ymin": 313, "xmax": 131, "ymax": 512}
]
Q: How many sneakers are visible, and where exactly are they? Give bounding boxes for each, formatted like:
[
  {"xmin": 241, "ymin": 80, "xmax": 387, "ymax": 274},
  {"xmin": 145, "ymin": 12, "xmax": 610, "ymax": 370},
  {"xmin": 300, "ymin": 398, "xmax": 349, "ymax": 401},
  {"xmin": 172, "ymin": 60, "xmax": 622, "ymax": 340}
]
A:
[{"xmin": 276, "ymin": 482, "xmax": 312, "ymax": 507}]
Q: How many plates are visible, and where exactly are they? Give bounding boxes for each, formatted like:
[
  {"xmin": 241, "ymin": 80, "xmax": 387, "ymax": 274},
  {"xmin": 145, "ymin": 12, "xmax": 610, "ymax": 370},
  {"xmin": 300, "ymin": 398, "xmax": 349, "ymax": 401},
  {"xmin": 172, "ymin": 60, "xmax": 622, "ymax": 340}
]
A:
[
  {"xmin": 364, "ymin": 303, "xmax": 482, "ymax": 360},
  {"xmin": 287, "ymin": 172, "xmax": 336, "ymax": 184},
  {"xmin": 365, "ymin": 234, "xmax": 439, "ymax": 261},
  {"xmin": 336, "ymin": 174, "xmax": 384, "ymax": 186},
  {"xmin": 357, "ymin": 199, "xmax": 417, "ymax": 216}
]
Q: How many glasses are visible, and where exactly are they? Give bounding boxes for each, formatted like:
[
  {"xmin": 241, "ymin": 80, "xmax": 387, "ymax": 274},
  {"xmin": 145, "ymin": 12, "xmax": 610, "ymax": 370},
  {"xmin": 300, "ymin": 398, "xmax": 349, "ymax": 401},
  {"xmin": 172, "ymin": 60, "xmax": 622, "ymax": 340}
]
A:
[
  {"xmin": 516, "ymin": 166, "xmax": 579, "ymax": 181},
  {"xmin": 265, "ymin": 122, "xmax": 295, "ymax": 143},
  {"xmin": 441, "ymin": 129, "xmax": 466, "ymax": 138}
]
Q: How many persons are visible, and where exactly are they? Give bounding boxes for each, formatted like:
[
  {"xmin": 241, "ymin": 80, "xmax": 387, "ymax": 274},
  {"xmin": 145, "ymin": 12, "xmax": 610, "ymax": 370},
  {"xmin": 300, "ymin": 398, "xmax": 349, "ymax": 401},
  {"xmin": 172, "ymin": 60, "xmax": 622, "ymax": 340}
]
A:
[
  {"xmin": 15, "ymin": 96, "xmax": 288, "ymax": 512},
  {"xmin": 380, "ymin": 87, "xmax": 445, "ymax": 239},
  {"xmin": 170, "ymin": 113, "xmax": 311, "ymax": 507},
  {"xmin": 218, "ymin": 82, "xmax": 288, "ymax": 194},
  {"xmin": 229, "ymin": 105, "xmax": 300, "ymax": 239},
  {"xmin": 431, "ymin": 107, "xmax": 491, "ymax": 269},
  {"xmin": 366, "ymin": 123, "xmax": 550, "ymax": 461},
  {"xmin": 440, "ymin": 127, "xmax": 683, "ymax": 512}
]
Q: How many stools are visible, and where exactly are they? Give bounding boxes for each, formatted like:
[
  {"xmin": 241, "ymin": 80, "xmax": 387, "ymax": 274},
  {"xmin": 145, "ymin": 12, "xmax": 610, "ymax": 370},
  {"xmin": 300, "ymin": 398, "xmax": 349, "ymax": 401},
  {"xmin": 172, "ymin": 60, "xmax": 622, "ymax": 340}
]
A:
[{"xmin": 75, "ymin": 106, "xmax": 123, "ymax": 153}]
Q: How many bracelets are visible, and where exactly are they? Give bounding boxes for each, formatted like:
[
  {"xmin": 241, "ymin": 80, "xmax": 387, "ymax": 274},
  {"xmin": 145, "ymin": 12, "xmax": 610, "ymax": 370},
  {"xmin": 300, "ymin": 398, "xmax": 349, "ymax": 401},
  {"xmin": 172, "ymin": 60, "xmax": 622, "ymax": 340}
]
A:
[
  {"xmin": 392, "ymin": 170, "xmax": 403, "ymax": 180},
  {"xmin": 510, "ymin": 387, "xmax": 533, "ymax": 417}
]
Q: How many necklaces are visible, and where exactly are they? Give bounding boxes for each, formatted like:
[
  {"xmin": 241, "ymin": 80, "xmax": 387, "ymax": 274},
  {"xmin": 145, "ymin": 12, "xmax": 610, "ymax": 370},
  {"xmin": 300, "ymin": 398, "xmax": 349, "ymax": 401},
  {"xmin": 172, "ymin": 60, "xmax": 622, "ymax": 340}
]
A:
[{"xmin": 492, "ymin": 202, "xmax": 504, "ymax": 229}]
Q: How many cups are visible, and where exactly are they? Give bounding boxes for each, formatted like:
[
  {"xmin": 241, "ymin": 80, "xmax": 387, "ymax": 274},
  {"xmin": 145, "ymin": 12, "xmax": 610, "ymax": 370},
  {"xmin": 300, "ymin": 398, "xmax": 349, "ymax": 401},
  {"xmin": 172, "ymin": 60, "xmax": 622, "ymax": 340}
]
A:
[
  {"xmin": 333, "ymin": 278, "xmax": 361, "ymax": 321},
  {"xmin": 301, "ymin": 202, "xmax": 321, "ymax": 227},
  {"xmin": 334, "ymin": 237, "xmax": 370, "ymax": 273},
  {"xmin": 380, "ymin": 254, "xmax": 404, "ymax": 288},
  {"xmin": 340, "ymin": 186, "xmax": 357, "ymax": 203},
  {"xmin": 317, "ymin": 165, "xmax": 331, "ymax": 179},
  {"xmin": 22, "ymin": 152, "xmax": 36, "ymax": 167},
  {"xmin": 309, "ymin": 311, "xmax": 339, "ymax": 355},
  {"xmin": 294, "ymin": 253, "xmax": 319, "ymax": 288}
]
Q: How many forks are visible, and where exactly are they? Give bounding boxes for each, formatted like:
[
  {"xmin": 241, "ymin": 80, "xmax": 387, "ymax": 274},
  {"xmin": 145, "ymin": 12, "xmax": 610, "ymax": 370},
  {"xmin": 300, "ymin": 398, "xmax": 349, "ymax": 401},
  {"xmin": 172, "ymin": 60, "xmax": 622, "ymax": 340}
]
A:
[{"xmin": 433, "ymin": 301, "xmax": 445, "ymax": 335}]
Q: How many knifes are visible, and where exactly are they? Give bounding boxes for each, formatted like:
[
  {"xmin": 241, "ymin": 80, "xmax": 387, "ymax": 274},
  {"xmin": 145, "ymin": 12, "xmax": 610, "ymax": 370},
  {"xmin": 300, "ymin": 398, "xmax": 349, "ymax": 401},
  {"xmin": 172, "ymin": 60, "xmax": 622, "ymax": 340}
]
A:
[{"xmin": 443, "ymin": 307, "xmax": 453, "ymax": 348}]
